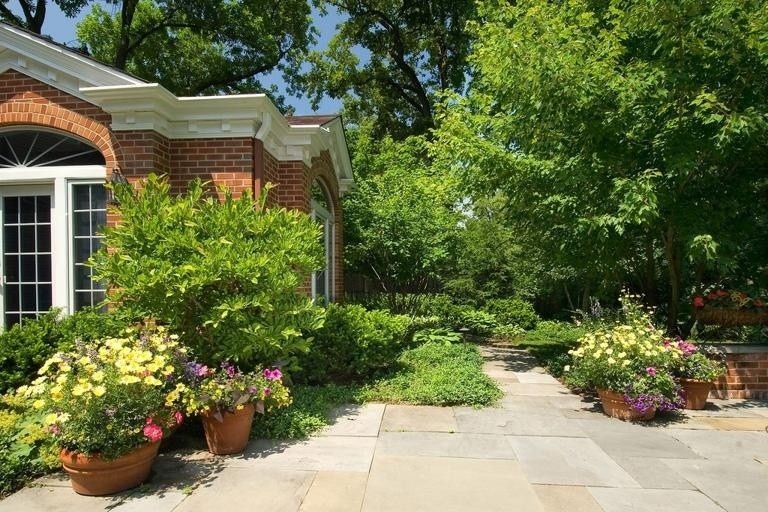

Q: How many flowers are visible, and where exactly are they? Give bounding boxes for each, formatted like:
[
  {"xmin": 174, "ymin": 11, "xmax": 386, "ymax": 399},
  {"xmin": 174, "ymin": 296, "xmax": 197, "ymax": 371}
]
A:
[
  {"xmin": 563, "ymin": 324, "xmax": 698, "ymax": 417},
  {"xmin": 192, "ymin": 366, "xmax": 293, "ymax": 418},
  {"xmin": 8, "ymin": 338, "xmax": 221, "ymax": 460},
  {"xmin": 668, "ymin": 341, "xmax": 726, "ymax": 381},
  {"xmin": 694, "ymin": 291, "xmax": 766, "ymax": 312}
]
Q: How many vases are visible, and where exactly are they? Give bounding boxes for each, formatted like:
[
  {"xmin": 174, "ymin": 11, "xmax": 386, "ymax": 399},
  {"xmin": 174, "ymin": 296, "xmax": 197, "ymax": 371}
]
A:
[
  {"xmin": 202, "ymin": 403, "xmax": 256, "ymax": 455},
  {"xmin": 668, "ymin": 377, "xmax": 712, "ymax": 411},
  {"xmin": 598, "ymin": 387, "xmax": 657, "ymax": 421},
  {"xmin": 697, "ymin": 306, "xmax": 766, "ymax": 328},
  {"xmin": 61, "ymin": 440, "xmax": 163, "ymax": 496}
]
